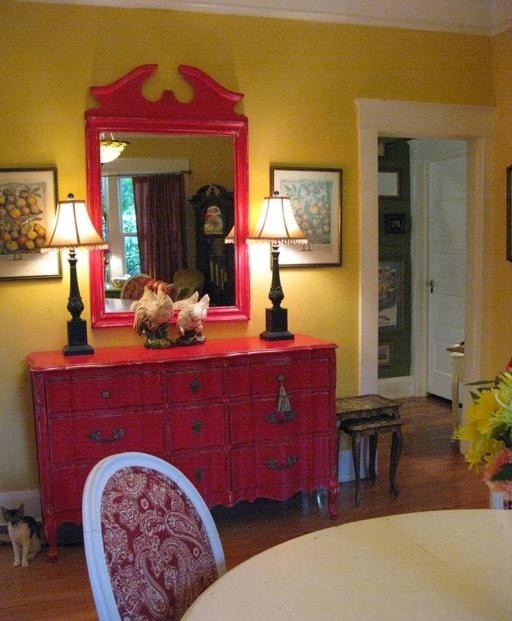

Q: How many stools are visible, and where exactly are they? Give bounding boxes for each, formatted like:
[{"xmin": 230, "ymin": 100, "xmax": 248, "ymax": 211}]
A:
[
  {"xmin": 342, "ymin": 414, "xmax": 409, "ymax": 504},
  {"xmin": 335, "ymin": 393, "xmax": 404, "ymax": 496}
]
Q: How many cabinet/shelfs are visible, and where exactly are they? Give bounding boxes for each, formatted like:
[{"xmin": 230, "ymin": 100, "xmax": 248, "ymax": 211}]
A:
[
  {"xmin": 25, "ymin": 334, "xmax": 337, "ymax": 559},
  {"xmin": 188, "ymin": 185, "xmax": 235, "ymax": 307}
]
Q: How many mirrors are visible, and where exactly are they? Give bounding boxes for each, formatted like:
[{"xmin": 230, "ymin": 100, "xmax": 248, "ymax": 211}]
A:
[{"xmin": 82, "ymin": 64, "xmax": 253, "ymax": 329}]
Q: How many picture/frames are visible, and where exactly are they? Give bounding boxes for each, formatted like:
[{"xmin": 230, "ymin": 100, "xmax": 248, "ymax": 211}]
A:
[
  {"xmin": 1, "ymin": 167, "xmax": 63, "ymax": 281},
  {"xmin": 269, "ymin": 165, "xmax": 343, "ymax": 268}
]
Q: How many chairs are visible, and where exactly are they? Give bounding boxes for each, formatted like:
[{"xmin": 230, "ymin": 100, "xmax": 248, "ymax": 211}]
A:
[
  {"xmin": 121, "ymin": 268, "xmax": 206, "ymax": 300},
  {"xmin": 81, "ymin": 451, "xmax": 229, "ymax": 621}
]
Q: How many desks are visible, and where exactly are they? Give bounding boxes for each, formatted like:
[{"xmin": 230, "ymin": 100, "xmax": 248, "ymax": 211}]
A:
[{"xmin": 180, "ymin": 509, "xmax": 511, "ymax": 621}]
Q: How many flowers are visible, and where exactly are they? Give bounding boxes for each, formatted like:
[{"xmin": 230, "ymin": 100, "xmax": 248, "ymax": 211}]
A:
[{"xmin": 449, "ymin": 357, "xmax": 512, "ymax": 504}]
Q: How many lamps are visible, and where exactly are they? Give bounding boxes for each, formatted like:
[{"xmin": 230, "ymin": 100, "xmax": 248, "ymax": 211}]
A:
[
  {"xmin": 39, "ymin": 194, "xmax": 110, "ymax": 356},
  {"xmin": 246, "ymin": 191, "xmax": 308, "ymax": 341},
  {"xmin": 100, "ymin": 131, "xmax": 130, "ymax": 167}
]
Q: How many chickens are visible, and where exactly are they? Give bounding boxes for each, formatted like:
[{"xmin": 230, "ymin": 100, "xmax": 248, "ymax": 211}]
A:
[{"xmin": 130, "ymin": 280, "xmax": 210, "ymax": 344}]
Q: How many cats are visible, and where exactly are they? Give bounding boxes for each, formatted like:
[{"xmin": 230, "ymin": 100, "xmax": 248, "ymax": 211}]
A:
[{"xmin": 0, "ymin": 504, "xmax": 44, "ymax": 567}]
